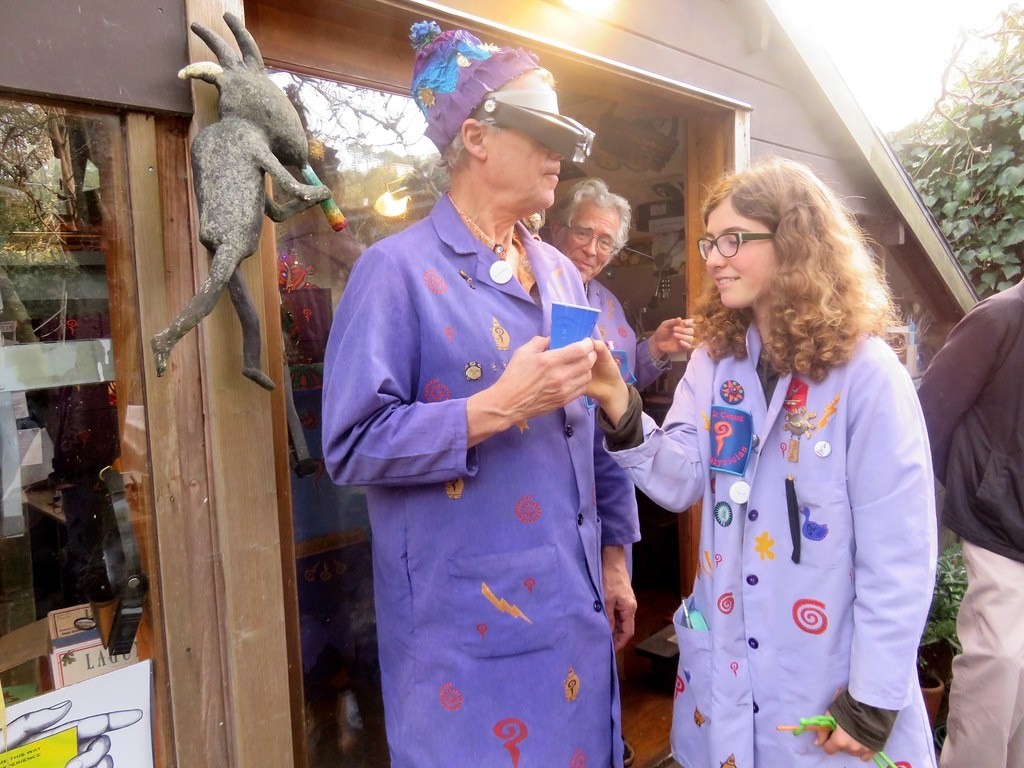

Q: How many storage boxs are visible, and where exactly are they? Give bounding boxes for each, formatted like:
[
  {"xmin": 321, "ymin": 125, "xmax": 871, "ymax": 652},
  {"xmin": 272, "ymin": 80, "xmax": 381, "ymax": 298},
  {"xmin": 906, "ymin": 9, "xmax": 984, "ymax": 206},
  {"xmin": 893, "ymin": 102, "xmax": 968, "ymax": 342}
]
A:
[{"xmin": 1, "ymin": 604, "xmax": 139, "ymax": 690}]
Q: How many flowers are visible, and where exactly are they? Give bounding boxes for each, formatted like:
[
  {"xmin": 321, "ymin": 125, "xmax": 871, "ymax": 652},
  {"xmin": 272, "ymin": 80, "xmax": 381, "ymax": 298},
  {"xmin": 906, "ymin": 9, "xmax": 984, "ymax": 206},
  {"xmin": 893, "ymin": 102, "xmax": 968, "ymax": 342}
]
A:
[
  {"xmin": 409, "ymin": 20, "xmax": 436, "ymax": 49},
  {"xmin": 418, "ymin": 88, "xmax": 435, "ymax": 109},
  {"xmin": 477, "ymin": 42, "xmax": 502, "ymax": 52}
]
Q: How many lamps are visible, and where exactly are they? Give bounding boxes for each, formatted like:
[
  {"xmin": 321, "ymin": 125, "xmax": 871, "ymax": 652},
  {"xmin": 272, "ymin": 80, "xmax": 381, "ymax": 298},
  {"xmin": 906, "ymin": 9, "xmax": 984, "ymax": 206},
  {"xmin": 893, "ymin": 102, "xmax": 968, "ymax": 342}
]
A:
[{"xmin": 374, "ymin": 158, "xmax": 448, "ymax": 223}]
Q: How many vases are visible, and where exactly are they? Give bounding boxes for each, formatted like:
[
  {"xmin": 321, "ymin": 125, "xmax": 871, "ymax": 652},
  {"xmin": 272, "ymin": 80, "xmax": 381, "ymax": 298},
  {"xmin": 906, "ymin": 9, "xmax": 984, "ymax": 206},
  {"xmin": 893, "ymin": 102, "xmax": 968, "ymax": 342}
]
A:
[{"xmin": 919, "ymin": 672, "xmax": 944, "ymax": 732}]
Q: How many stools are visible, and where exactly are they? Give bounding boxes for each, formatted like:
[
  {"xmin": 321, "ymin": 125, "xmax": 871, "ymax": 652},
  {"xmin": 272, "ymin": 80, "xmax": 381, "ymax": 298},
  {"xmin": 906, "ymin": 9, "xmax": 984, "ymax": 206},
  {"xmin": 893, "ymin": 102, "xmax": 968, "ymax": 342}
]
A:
[{"xmin": 636, "ymin": 624, "xmax": 680, "ymax": 695}]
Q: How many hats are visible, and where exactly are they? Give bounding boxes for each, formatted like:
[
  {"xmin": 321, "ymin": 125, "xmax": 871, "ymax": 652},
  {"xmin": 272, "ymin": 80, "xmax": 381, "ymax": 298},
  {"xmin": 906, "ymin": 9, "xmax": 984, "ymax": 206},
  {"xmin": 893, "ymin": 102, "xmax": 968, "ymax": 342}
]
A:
[{"xmin": 409, "ymin": 19, "xmax": 543, "ymax": 155}]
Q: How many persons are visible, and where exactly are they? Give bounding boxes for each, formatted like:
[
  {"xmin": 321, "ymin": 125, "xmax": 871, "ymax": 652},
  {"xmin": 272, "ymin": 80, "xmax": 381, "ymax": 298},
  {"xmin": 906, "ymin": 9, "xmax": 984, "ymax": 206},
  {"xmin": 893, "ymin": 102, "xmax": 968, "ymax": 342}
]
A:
[
  {"xmin": 587, "ymin": 159, "xmax": 939, "ymax": 768},
  {"xmin": 291, "ymin": 465, "xmax": 377, "ymax": 681},
  {"xmin": 548, "ymin": 167, "xmax": 696, "ymax": 765},
  {"xmin": 320, "ymin": 20, "xmax": 642, "ymax": 768},
  {"xmin": 920, "ymin": 277, "xmax": 1024, "ymax": 768},
  {"xmin": 25, "ymin": 315, "xmax": 120, "ymax": 607}
]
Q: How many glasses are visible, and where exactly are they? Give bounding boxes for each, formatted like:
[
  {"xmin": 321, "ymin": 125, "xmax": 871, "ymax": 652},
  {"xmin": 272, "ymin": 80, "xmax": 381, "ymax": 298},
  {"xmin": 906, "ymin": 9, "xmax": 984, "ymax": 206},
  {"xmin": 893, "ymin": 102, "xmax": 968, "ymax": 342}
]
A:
[
  {"xmin": 697, "ymin": 232, "xmax": 775, "ymax": 261},
  {"xmin": 473, "ymin": 97, "xmax": 595, "ymax": 162},
  {"xmin": 562, "ymin": 223, "xmax": 619, "ymax": 255}
]
